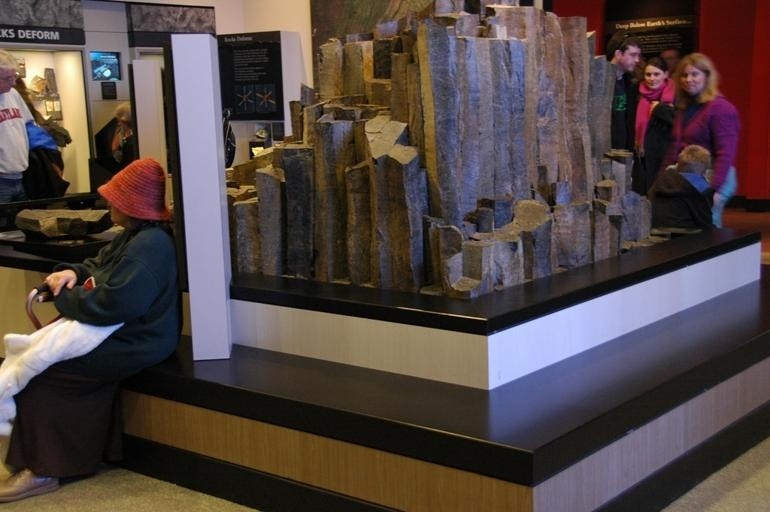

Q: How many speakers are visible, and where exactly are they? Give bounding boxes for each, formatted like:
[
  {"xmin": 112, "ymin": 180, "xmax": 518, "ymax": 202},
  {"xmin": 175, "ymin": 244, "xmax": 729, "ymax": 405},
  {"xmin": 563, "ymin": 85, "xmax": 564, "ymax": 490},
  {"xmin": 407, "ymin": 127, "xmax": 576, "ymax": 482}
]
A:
[{"xmin": 102, "ymin": 82, "xmax": 117, "ymax": 99}]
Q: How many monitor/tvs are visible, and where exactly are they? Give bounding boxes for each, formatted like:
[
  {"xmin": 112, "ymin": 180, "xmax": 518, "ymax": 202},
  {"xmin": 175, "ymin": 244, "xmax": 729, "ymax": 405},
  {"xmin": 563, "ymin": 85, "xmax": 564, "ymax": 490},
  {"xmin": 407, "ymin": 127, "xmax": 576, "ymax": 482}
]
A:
[{"xmin": 89, "ymin": 52, "xmax": 122, "ymax": 82}]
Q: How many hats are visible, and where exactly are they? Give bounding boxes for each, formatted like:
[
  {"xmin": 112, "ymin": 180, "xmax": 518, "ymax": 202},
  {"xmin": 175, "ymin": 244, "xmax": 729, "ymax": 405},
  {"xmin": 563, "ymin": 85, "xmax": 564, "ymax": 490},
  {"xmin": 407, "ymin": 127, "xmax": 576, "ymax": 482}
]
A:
[{"xmin": 97, "ymin": 158, "xmax": 172, "ymax": 222}]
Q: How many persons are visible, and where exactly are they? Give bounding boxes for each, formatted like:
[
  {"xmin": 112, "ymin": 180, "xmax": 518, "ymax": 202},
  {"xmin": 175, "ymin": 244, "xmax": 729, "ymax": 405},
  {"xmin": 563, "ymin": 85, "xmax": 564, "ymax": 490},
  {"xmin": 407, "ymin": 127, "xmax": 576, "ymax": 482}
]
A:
[
  {"xmin": 0, "ymin": 49, "xmax": 71, "ymax": 206},
  {"xmin": 0, "ymin": 158, "xmax": 183, "ymax": 506},
  {"xmin": 113, "ymin": 103, "xmax": 135, "ymax": 163},
  {"xmin": 605, "ymin": 27, "xmax": 740, "ymax": 226}
]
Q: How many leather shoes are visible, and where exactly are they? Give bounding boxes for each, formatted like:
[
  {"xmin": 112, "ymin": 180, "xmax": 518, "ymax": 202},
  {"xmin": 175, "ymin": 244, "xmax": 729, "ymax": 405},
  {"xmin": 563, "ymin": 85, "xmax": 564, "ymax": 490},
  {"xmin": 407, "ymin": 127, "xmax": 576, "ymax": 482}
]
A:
[{"xmin": 0, "ymin": 468, "xmax": 60, "ymax": 503}]
